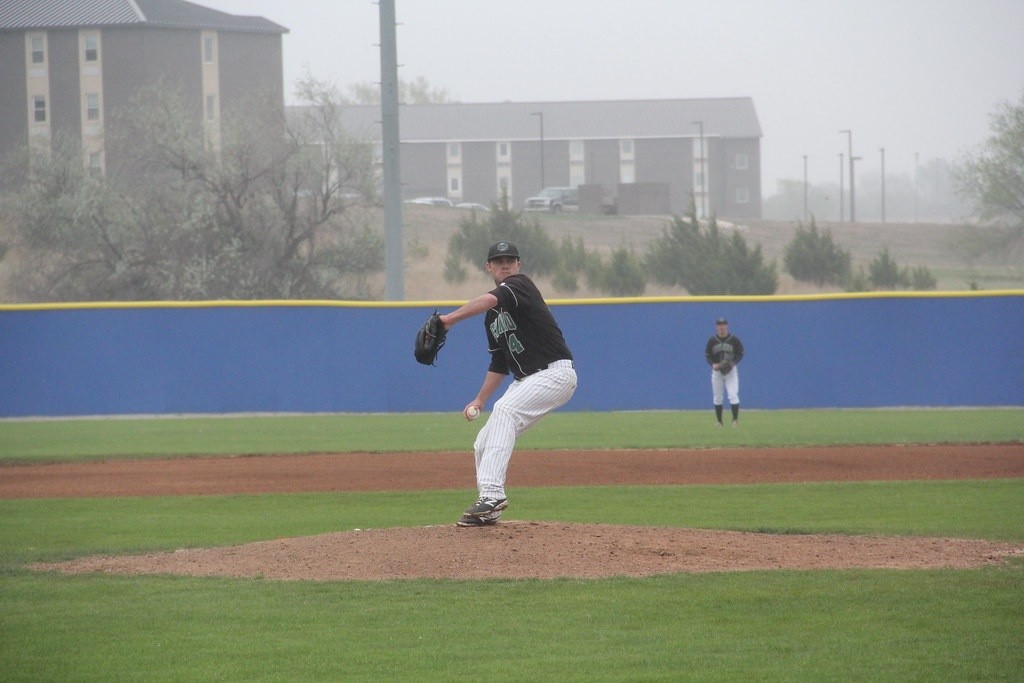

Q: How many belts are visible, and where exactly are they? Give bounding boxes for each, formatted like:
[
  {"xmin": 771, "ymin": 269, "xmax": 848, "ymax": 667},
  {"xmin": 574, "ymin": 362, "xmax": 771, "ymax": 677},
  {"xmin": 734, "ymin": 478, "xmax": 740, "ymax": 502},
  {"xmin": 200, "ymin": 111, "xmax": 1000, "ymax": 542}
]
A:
[{"xmin": 534, "ymin": 364, "xmax": 549, "ymax": 373}]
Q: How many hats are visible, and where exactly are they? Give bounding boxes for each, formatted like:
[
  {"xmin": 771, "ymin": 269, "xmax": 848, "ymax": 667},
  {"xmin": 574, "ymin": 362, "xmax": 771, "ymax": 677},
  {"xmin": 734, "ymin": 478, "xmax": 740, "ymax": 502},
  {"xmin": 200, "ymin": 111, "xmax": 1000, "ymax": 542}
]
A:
[
  {"xmin": 487, "ymin": 241, "xmax": 521, "ymax": 262},
  {"xmin": 715, "ymin": 318, "xmax": 728, "ymax": 326}
]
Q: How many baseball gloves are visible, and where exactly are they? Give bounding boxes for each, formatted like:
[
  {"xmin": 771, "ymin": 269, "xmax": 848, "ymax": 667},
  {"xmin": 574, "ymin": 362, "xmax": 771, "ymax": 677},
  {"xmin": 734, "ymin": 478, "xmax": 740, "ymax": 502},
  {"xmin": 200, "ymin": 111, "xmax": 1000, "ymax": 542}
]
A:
[
  {"xmin": 415, "ymin": 312, "xmax": 448, "ymax": 365},
  {"xmin": 719, "ymin": 361, "xmax": 733, "ymax": 375}
]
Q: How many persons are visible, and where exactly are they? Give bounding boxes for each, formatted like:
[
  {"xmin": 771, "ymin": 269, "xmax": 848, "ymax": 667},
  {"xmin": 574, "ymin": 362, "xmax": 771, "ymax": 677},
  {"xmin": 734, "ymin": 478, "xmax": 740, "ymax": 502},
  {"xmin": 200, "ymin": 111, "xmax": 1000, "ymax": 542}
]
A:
[
  {"xmin": 705, "ymin": 319, "xmax": 743, "ymax": 428},
  {"xmin": 414, "ymin": 242, "xmax": 578, "ymax": 527}
]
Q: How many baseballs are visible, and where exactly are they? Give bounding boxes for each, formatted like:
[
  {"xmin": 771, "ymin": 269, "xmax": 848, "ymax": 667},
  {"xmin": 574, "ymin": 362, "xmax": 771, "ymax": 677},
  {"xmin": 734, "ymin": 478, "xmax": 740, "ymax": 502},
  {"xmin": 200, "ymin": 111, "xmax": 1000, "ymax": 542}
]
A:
[{"xmin": 466, "ymin": 406, "xmax": 480, "ymax": 420}]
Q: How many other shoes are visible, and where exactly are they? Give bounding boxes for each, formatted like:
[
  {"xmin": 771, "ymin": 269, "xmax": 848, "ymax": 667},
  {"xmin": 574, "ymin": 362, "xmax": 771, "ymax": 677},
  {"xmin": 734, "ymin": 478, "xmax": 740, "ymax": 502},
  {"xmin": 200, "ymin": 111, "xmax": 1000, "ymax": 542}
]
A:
[
  {"xmin": 731, "ymin": 418, "xmax": 740, "ymax": 426},
  {"xmin": 716, "ymin": 422, "xmax": 724, "ymax": 428}
]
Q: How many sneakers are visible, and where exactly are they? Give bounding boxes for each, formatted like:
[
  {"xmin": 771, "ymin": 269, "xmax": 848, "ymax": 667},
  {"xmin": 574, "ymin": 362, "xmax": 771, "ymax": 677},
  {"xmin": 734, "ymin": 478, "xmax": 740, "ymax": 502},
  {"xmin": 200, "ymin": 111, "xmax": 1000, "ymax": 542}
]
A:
[
  {"xmin": 460, "ymin": 496, "xmax": 509, "ymax": 520},
  {"xmin": 457, "ymin": 514, "xmax": 505, "ymax": 528}
]
